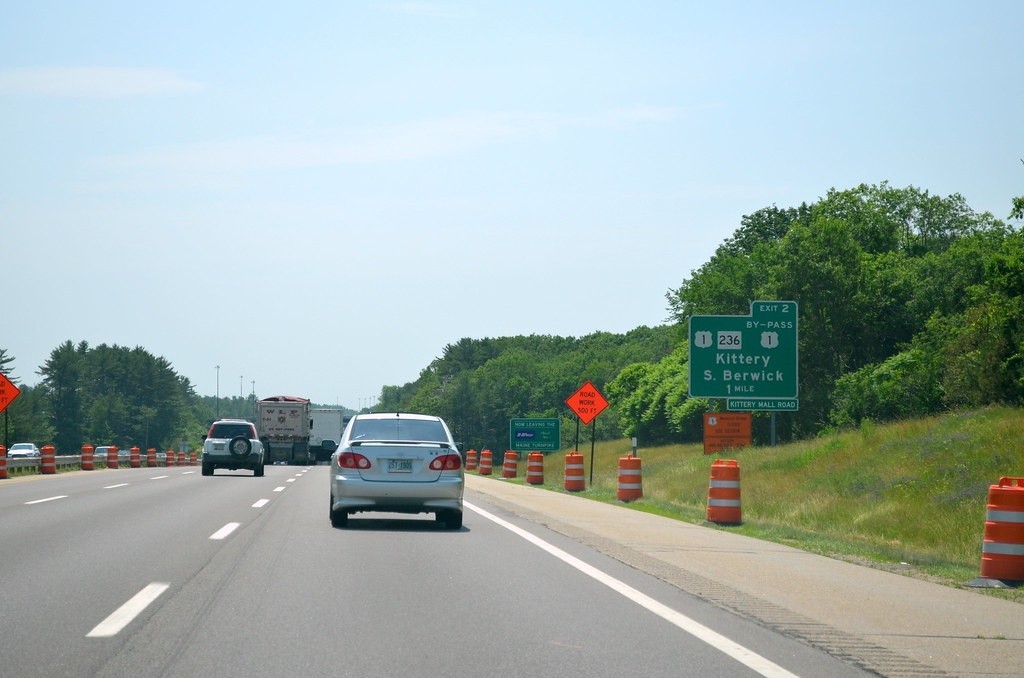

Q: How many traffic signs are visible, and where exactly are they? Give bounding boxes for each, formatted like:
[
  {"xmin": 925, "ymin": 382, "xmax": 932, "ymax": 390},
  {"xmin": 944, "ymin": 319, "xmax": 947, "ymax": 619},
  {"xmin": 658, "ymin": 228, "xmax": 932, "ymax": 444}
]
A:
[{"xmin": 689, "ymin": 300, "xmax": 799, "ymax": 412}]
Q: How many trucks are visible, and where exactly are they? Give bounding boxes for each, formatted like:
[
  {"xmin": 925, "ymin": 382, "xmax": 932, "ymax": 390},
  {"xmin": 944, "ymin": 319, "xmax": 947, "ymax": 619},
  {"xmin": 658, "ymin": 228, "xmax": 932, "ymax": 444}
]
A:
[
  {"xmin": 310, "ymin": 408, "xmax": 342, "ymax": 464},
  {"xmin": 257, "ymin": 396, "xmax": 314, "ymax": 465}
]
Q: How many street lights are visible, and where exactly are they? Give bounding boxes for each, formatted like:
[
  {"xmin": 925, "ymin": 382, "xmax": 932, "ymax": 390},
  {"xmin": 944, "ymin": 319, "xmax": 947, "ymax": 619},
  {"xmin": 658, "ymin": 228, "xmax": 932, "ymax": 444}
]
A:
[
  {"xmin": 215, "ymin": 365, "xmax": 220, "ymax": 418},
  {"xmin": 239, "ymin": 375, "xmax": 243, "ymax": 417},
  {"xmin": 251, "ymin": 381, "xmax": 256, "ymax": 418}
]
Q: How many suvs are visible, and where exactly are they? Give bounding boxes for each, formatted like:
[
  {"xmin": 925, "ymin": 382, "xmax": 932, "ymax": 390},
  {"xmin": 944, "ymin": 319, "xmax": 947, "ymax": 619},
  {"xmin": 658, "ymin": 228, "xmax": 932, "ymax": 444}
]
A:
[{"xmin": 201, "ymin": 419, "xmax": 268, "ymax": 476}]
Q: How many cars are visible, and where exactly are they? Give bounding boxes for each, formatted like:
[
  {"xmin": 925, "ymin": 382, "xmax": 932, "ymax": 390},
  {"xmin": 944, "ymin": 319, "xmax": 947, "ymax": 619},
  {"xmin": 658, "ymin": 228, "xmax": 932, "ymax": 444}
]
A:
[
  {"xmin": 8, "ymin": 443, "xmax": 40, "ymax": 458},
  {"xmin": 321, "ymin": 411, "xmax": 467, "ymax": 529},
  {"xmin": 94, "ymin": 446, "xmax": 110, "ymax": 456}
]
